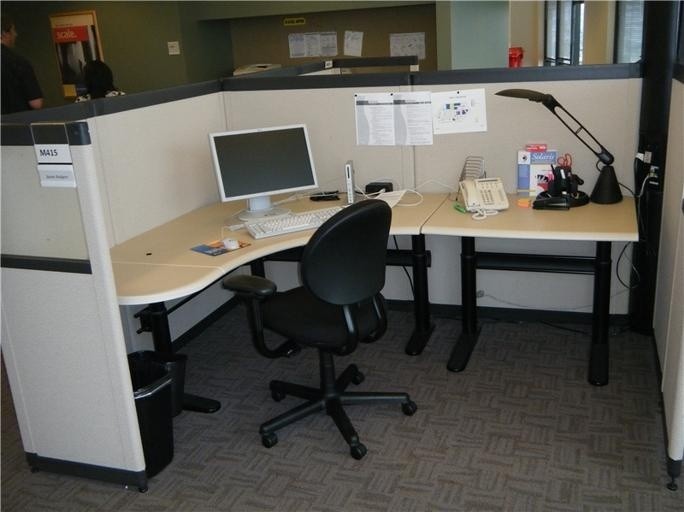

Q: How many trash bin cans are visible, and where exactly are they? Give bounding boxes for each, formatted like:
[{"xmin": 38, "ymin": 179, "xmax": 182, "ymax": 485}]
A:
[{"xmin": 127, "ymin": 350, "xmax": 176, "ymax": 480}]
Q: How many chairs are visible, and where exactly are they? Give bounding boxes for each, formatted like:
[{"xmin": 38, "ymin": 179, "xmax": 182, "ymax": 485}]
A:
[{"xmin": 221, "ymin": 199, "xmax": 418, "ymax": 462}]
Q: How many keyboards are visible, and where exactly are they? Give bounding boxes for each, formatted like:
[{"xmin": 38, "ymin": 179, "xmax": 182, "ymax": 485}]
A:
[{"xmin": 244, "ymin": 205, "xmax": 343, "ymax": 240}]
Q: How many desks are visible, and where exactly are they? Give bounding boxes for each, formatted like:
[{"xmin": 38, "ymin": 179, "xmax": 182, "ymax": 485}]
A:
[
  {"xmin": 421, "ymin": 192, "xmax": 640, "ymax": 389},
  {"xmin": 108, "ymin": 192, "xmax": 452, "ymax": 414}
]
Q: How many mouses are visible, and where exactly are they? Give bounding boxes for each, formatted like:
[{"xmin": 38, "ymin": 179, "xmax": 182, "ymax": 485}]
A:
[{"xmin": 222, "ymin": 237, "xmax": 241, "ymax": 251}]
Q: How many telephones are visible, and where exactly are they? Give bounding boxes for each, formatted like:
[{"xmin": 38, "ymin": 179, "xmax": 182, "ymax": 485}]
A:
[{"xmin": 459, "ymin": 177, "xmax": 510, "ymax": 220}]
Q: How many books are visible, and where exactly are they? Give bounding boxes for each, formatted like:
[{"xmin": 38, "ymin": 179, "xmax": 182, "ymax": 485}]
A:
[{"xmin": 517, "ymin": 150, "xmax": 558, "ymax": 196}]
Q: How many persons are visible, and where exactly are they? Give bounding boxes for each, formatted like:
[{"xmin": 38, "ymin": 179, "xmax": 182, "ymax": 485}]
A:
[
  {"xmin": 75, "ymin": 60, "xmax": 125, "ymax": 103},
  {"xmin": 0, "ymin": 12, "xmax": 43, "ymax": 115}
]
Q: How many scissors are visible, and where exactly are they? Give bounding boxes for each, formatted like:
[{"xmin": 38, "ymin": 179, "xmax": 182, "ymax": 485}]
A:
[{"xmin": 557, "ymin": 153, "xmax": 572, "ymax": 166}]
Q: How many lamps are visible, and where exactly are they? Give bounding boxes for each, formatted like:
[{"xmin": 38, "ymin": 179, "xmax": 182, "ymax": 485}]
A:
[{"xmin": 495, "ymin": 89, "xmax": 623, "ymax": 204}]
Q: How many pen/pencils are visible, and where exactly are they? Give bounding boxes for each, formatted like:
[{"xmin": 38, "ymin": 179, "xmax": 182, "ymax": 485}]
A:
[{"xmin": 550, "ymin": 163, "xmax": 571, "ymax": 179}]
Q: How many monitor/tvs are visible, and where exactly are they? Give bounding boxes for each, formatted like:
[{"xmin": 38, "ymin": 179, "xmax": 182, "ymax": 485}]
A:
[{"xmin": 207, "ymin": 122, "xmax": 320, "ymax": 223}]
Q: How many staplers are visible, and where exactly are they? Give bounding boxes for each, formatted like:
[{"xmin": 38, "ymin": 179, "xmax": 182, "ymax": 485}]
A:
[{"xmin": 532, "ymin": 197, "xmax": 569, "ymax": 210}]
